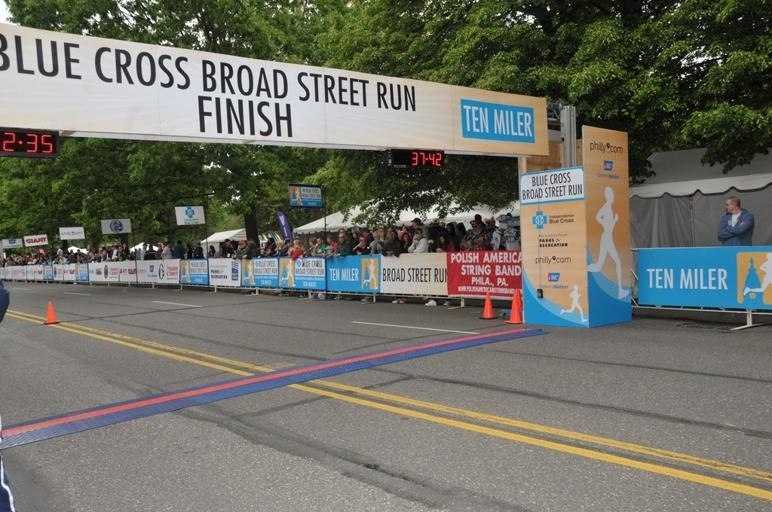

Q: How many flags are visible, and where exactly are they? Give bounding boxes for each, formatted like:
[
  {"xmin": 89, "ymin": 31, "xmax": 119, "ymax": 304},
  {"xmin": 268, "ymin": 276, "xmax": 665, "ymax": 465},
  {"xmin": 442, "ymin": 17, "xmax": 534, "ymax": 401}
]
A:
[{"xmin": 275, "ymin": 212, "xmax": 293, "ymax": 241}]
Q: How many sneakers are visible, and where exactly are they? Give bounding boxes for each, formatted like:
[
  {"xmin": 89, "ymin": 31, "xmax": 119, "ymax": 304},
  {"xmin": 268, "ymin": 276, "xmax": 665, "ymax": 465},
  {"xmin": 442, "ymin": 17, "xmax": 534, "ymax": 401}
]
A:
[
  {"xmin": 392, "ymin": 298, "xmax": 407, "ymax": 303},
  {"xmin": 425, "ymin": 299, "xmax": 437, "ymax": 307},
  {"xmin": 444, "ymin": 301, "xmax": 452, "ymax": 306},
  {"xmin": 361, "ymin": 296, "xmax": 372, "ymax": 302}
]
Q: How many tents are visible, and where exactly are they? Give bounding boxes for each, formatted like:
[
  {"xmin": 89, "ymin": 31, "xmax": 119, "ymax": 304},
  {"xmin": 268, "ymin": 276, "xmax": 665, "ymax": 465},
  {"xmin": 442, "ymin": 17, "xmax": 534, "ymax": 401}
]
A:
[
  {"xmin": 294, "ymin": 187, "xmax": 521, "ymax": 235},
  {"xmin": 201, "ymin": 228, "xmax": 248, "ymax": 257}
]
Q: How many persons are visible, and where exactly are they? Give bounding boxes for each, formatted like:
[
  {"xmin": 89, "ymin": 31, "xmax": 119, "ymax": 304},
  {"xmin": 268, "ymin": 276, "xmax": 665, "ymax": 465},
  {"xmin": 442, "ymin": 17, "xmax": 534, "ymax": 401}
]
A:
[
  {"xmin": 208, "ymin": 237, "xmax": 325, "ymax": 300},
  {"xmin": 54, "ymin": 244, "xmax": 136, "ymax": 287},
  {"xmin": 137, "ymin": 239, "xmax": 204, "ymax": 260},
  {"xmin": 717, "ymin": 196, "xmax": 754, "ymax": 246},
  {"xmin": 325, "ymin": 212, "xmax": 521, "ymax": 306},
  {"xmin": 2, "ymin": 249, "xmax": 54, "ymax": 284}
]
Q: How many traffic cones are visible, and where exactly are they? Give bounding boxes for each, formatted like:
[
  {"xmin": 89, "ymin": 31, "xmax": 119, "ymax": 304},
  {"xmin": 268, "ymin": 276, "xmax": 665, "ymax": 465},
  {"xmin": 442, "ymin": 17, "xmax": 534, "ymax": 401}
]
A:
[
  {"xmin": 506, "ymin": 289, "xmax": 523, "ymax": 325},
  {"xmin": 480, "ymin": 292, "xmax": 500, "ymax": 320},
  {"xmin": 44, "ymin": 300, "xmax": 60, "ymax": 326}
]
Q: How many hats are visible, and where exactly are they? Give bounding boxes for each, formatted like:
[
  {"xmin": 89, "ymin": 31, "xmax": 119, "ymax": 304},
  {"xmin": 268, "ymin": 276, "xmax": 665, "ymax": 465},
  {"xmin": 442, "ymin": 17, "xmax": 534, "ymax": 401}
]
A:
[
  {"xmin": 411, "ymin": 218, "xmax": 421, "ymax": 223},
  {"xmin": 238, "ymin": 240, "xmax": 245, "ymax": 245}
]
